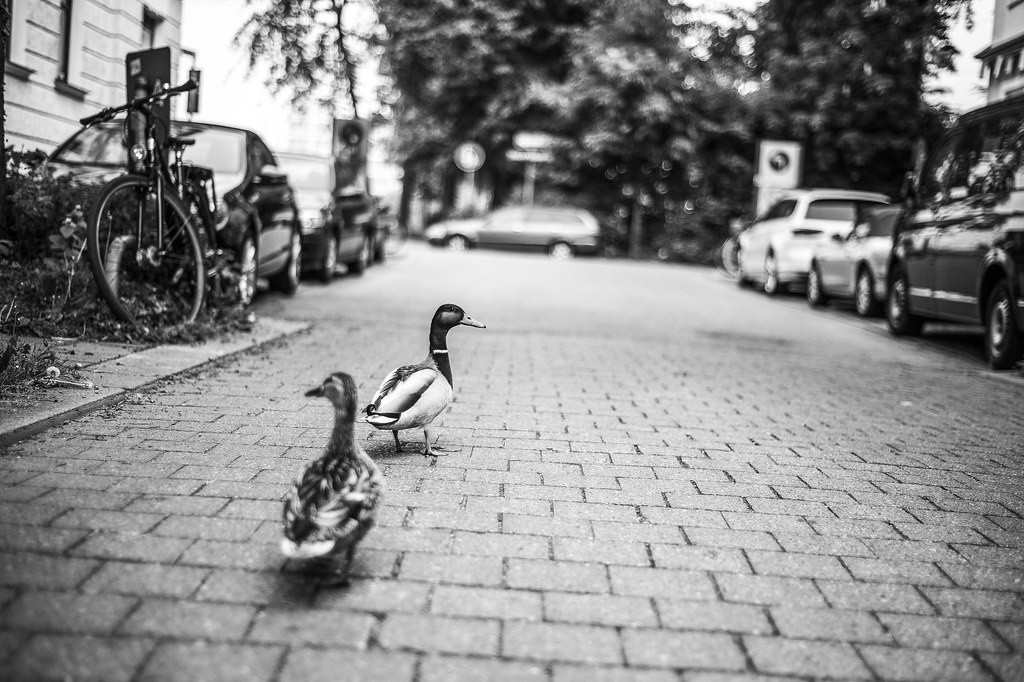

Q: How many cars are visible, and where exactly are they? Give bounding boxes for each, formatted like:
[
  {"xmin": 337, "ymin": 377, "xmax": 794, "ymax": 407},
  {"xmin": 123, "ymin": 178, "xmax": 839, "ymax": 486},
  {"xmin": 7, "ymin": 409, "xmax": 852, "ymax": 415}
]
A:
[
  {"xmin": 886, "ymin": 151, "xmax": 1023, "ymax": 369},
  {"xmin": 36, "ymin": 120, "xmax": 303, "ymax": 307},
  {"xmin": 423, "ymin": 207, "xmax": 601, "ymax": 261},
  {"xmin": 805, "ymin": 202, "xmax": 901, "ymax": 318},
  {"xmin": 270, "ymin": 152, "xmax": 390, "ymax": 283},
  {"xmin": 729, "ymin": 187, "xmax": 891, "ymax": 298}
]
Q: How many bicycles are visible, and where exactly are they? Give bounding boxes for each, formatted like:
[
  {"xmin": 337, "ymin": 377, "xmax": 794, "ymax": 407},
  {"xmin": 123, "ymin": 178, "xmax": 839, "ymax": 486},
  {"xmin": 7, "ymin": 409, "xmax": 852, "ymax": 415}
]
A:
[{"xmin": 75, "ymin": 69, "xmax": 270, "ymax": 336}]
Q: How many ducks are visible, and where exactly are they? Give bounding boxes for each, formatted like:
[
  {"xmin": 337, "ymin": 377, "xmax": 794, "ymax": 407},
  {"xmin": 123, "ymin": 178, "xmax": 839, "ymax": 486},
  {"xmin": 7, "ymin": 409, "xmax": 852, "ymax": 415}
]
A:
[
  {"xmin": 280, "ymin": 371, "xmax": 385, "ymax": 586},
  {"xmin": 355, "ymin": 304, "xmax": 486, "ymax": 456}
]
